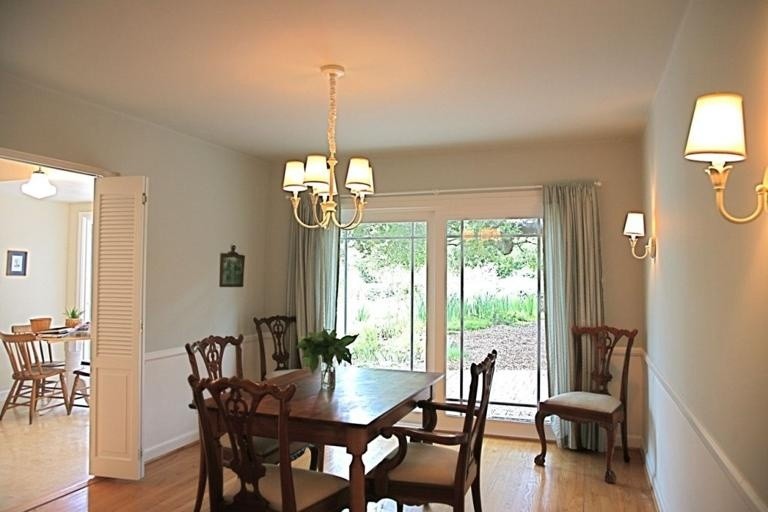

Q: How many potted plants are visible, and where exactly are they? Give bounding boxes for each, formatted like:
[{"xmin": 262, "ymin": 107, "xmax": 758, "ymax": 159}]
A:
[
  {"xmin": 297, "ymin": 327, "xmax": 359, "ymax": 390},
  {"xmin": 64, "ymin": 306, "xmax": 85, "ymax": 328}
]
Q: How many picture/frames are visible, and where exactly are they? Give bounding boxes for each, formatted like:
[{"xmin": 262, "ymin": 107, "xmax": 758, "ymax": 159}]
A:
[
  {"xmin": 6, "ymin": 250, "xmax": 27, "ymax": 276},
  {"xmin": 219, "ymin": 252, "xmax": 245, "ymax": 287}
]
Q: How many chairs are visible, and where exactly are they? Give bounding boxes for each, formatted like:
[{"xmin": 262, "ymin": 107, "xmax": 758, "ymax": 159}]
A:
[
  {"xmin": 534, "ymin": 324, "xmax": 638, "ymax": 484},
  {"xmin": 253, "ymin": 315, "xmax": 318, "ymax": 473},
  {"xmin": 187, "ymin": 373, "xmax": 350, "ymax": 512},
  {"xmin": 0, "ymin": 318, "xmax": 90, "ymax": 426},
  {"xmin": 184, "ymin": 334, "xmax": 318, "ymax": 512},
  {"xmin": 364, "ymin": 349, "xmax": 496, "ymax": 512}
]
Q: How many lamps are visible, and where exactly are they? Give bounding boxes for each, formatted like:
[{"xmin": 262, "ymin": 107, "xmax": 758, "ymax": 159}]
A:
[
  {"xmin": 682, "ymin": 91, "xmax": 768, "ymax": 223},
  {"xmin": 21, "ymin": 165, "xmax": 57, "ymax": 200},
  {"xmin": 622, "ymin": 212, "xmax": 656, "ymax": 259},
  {"xmin": 282, "ymin": 64, "xmax": 374, "ymax": 230}
]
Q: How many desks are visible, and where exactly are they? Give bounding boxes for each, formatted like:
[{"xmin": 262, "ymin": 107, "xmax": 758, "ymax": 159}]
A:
[{"xmin": 189, "ymin": 364, "xmax": 446, "ymax": 512}]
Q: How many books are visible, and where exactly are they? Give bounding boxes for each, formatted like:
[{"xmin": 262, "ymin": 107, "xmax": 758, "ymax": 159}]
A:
[{"xmin": 36, "ymin": 327, "xmax": 74, "ymax": 338}]
